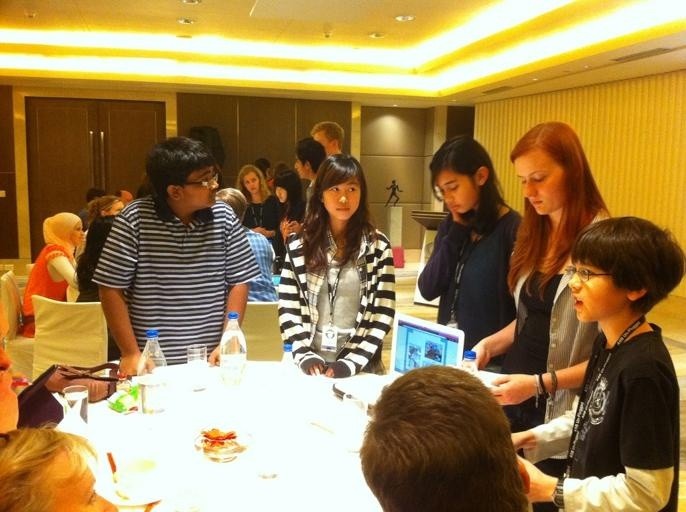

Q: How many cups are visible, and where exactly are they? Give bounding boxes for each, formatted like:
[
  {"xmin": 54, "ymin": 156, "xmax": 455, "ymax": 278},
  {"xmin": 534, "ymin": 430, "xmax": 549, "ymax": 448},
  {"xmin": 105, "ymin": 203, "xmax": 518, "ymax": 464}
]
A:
[
  {"xmin": 340, "ymin": 395, "xmax": 367, "ymax": 461},
  {"xmin": 188, "ymin": 345, "xmax": 207, "ymax": 391},
  {"xmin": 63, "ymin": 384, "xmax": 89, "ymax": 424}
]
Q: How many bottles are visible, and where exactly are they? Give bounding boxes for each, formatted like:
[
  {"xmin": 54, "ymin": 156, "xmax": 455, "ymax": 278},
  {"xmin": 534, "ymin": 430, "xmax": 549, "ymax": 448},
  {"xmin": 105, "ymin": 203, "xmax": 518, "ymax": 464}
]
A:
[
  {"xmin": 218, "ymin": 313, "xmax": 248, "ymax": 404},
  {"xmin": 134, "ymin": 330, "xmax": 172, "ymax": 442},
  {"xmin": 461, "ymin": 350, "xmax": 478, "ymax": 378},
  {"xmin": 272, "ymin": 342, "xmax": 299, "ymax": 423}
]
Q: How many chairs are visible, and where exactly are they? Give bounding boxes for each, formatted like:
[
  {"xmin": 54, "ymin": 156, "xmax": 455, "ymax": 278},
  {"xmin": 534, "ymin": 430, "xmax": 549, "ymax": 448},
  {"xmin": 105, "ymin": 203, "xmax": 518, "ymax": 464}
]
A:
[
  {"xmin": 240, "ymin": 303, "xmax": 283, "ymax": 361},
  {"xmin": 0, "ymin": 270, "xmax": 32, "ymax": 381},
  {"xmin": 31, "ymin": 295, "xmax": 107, "ymax": 382}
]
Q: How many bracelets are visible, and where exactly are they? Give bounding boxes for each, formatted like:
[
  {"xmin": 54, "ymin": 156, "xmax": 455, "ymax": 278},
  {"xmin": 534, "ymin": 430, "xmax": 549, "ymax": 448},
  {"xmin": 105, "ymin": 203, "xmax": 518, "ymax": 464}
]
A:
[{"xmin": 535, "ymin": 374, "xmax": 542, "ymax": 408}]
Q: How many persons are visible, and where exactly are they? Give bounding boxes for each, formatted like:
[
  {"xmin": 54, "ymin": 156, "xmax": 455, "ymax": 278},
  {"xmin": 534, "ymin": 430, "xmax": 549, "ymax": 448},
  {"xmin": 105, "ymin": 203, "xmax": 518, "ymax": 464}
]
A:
[
  {"xmin": 274, "ymin": 171, "xmax": 305, "ymax": 274},
  {"xmin": 214, "ymin": 189, "xmax": 279, "ymax": 302},
  {"xmin": 278, "ymin": 155, "xmax": 395, "ymax": 378},
  {"xmin": 237, "ymin": 165, "xmax": 281, "ymax": 257},
  {"xmin": 510, "ymin": 217, "xmax": 686, "ymax": 512},
  {"xmin": 469, "ymin": 121, "xmax": 619, "ymax": 512},
  {"xmin": 21, "ymin": 212, "xmax": 84, "ymax": 337},
  {"xmin": 311, "ymin": 122, "xmax": 344, "ymax": 157},
  {"xmin": 77, "ymin": 214, "xmax": 127, "ymax": 359},
  {"xmin": 420, "ymin": 138, "xmax": 526, "ymax": 372},
  {"xmin": 257, "ymin": 157, "xmax": 270, "ymax": 180},
  {"xmin": 294, "ymin": 138, "xmax": 327, "ymax": 233},
  {"xmin": 92, "ymin": 137, "xmax": 262, "ymax": 379},
  {"xmin": 384, "ymin": 180, "xmax": 404, "ymax": 206},
  {"xmin": 79, "ymin": 196, "xmax": 124, "ymax": 223},
  {"xmin": 1, "ymin": 428, "xmax": 117, "ymax": 511},
  {"xmin": 281, "ymin": 200, "xmax": 306, "ymax": 250},
  {"xmin": 268, "ymin": 161, "xmax": 290, "ymax": 193},
  {"xmin": 360, "ymin": 365, "xmax": 531, "ymax": 512},
  {"xmin": 115, "ymin": 189, "xmax": 133, "ymax": 204}
]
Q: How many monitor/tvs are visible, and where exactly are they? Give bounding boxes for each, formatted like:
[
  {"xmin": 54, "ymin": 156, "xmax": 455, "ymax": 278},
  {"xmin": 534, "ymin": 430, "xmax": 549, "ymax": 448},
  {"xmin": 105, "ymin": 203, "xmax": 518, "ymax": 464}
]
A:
[{"xmin": 390, "ymin": 312, "xmax": 466, "ymax": 380}]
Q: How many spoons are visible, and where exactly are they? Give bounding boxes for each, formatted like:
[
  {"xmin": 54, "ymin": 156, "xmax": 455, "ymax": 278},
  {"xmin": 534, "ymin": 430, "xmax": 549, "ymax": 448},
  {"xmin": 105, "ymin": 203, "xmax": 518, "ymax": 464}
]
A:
[{"xmin": 106, "ymin": 452, "xmax": 127, "ymax": 502}]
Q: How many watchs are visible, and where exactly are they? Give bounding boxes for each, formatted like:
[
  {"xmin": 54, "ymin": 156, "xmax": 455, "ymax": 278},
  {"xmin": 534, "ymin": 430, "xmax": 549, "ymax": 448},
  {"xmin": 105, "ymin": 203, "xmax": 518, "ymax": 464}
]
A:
[{"xmin": 553, "ymin": 481, "xmax": 565, "ymax": 508}]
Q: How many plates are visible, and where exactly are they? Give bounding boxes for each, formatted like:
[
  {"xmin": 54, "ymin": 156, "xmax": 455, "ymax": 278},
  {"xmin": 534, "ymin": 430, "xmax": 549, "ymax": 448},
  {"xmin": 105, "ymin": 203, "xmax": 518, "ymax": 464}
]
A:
[
  {"xmin": 93, "ymin": 473, "xmax": 163, "ymax": 506},
  {"xmin": 194, "ymin": 430, "xmax": 249, "ymax": 461}
]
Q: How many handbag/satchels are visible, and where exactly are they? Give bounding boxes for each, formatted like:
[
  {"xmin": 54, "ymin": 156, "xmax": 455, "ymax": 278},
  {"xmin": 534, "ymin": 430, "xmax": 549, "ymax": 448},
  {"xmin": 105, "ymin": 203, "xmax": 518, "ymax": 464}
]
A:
[{"xmin": 16, "ymin": 363, "xmax": 120, "ymax": 429}]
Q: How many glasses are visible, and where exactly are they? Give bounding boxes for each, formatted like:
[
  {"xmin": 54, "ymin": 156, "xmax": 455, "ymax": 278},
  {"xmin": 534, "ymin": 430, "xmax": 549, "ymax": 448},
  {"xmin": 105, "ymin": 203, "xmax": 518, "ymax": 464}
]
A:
[
  {"xmin": 564, "ymin": 266, "xmax": 614, "ymax": 279},
  {"xmin": 168, "ymin": 173, "xmax": 220, "ymax": 188}
]
